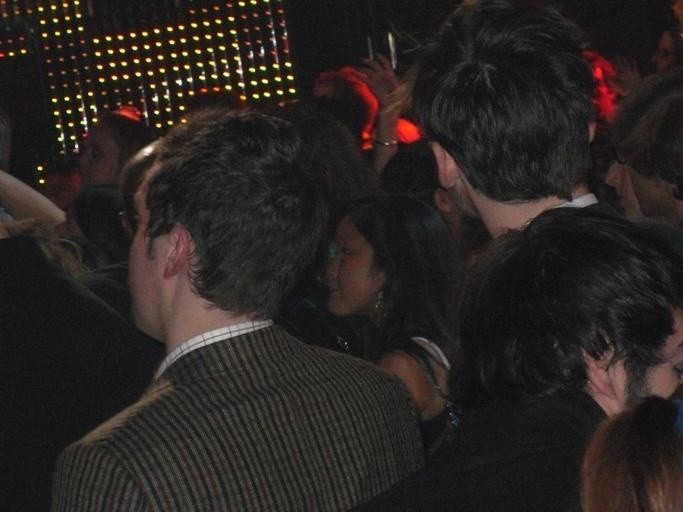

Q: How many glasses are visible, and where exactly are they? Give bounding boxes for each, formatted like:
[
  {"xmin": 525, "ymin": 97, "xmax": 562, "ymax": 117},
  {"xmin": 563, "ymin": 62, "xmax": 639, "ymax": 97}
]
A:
[{"xmin": 118, "ymin": 211, "xmax": 192, "ymax": 241}]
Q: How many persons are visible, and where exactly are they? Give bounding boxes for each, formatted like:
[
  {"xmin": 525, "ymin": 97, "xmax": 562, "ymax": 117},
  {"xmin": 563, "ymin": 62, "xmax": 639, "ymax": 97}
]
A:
[{"xmin": 0, "ymin": 1, "xmax": 682, "ymax": 511}]
